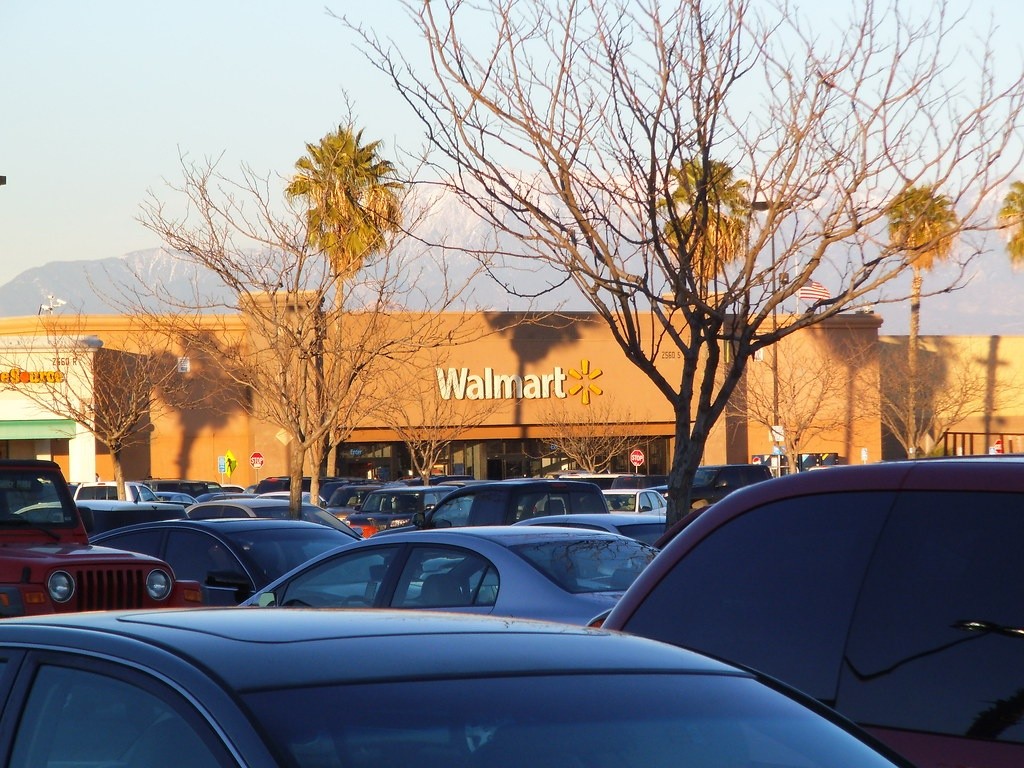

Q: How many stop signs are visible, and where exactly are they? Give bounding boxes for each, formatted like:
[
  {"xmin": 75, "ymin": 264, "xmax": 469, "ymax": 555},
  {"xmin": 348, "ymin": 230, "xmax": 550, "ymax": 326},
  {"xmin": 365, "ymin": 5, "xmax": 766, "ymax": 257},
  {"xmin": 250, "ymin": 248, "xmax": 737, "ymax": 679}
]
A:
[
  {"xmin": 631, "ymin": 451, "xmax": 644, "ymax": 466},
  {"xmin": 250, "ymin": 452, "xmax": 264, "ymax": 469}
]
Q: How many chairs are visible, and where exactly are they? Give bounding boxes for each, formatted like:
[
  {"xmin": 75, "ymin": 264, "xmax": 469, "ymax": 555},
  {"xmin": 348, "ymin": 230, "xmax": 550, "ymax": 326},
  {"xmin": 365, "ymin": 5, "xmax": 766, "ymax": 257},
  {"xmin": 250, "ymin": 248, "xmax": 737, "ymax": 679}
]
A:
[
  {"xmin": 544, "ymin": 499, "xmax": 565, "ymax": 516},
  {"xmin": 419, "ymin": 573, "xmax": 462, "ymax": 606},
  {"xmin": 348, "ymin": 496, "xmax": 358, "ymax": 507}
]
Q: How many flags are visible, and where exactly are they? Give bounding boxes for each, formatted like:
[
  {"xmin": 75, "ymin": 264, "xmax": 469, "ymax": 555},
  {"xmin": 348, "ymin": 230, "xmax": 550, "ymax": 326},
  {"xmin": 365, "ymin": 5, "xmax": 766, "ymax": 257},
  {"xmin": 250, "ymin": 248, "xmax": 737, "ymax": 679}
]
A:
[{"xmin": 799, "ymin": 277, "xmax": 830, "ymax": 300}]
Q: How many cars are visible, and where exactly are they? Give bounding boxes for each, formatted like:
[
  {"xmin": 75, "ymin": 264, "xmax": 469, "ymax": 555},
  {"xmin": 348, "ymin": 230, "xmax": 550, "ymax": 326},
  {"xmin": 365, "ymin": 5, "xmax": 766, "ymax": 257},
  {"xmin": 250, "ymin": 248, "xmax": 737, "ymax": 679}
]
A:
[{"xmin": 0, "ymin": 453, "xmax": 1024, "ymax": 768}]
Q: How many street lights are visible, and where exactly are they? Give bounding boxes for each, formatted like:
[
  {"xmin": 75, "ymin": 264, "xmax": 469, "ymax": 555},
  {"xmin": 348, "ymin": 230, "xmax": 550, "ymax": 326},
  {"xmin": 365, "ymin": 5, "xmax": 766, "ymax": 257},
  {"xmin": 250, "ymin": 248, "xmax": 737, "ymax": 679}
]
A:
[{"xmin": 755, "ymin": 203, "xmax": 793, "ymax": 478}]
